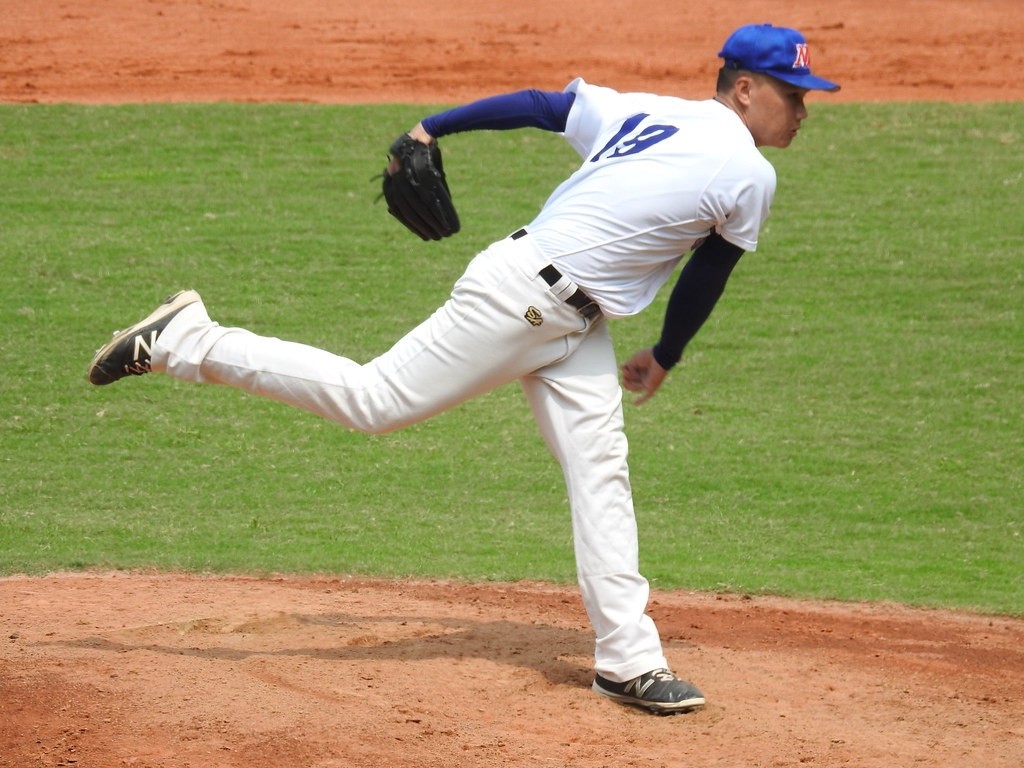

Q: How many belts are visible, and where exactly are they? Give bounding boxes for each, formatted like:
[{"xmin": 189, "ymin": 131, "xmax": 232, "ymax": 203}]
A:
[{"xmin": 511, "ymin": 229, "xmax": 601, "ymax": 317}]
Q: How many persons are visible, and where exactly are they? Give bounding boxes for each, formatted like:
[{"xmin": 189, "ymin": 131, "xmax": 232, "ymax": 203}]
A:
[{"xmin": 88, "ymin": 22, "xmax": 841, "ymax": 710}]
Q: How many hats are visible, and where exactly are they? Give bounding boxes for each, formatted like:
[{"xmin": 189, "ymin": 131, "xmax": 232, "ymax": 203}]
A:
[{"xmin": 718, "ymin": 24, "xmax": 841, "ymax": 92}]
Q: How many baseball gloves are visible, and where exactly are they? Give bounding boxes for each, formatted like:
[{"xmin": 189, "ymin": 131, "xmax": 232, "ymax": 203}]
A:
[{"xmin": 368, "ymin": 129, "xmax": 462, "ymax": 243}]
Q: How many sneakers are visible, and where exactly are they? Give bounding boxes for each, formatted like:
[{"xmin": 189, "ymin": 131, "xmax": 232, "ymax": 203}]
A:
[
  {"xmin": 88, "ymin": 289, "xmax": 201, "ymax": 386},
  {"xmin": 591, "ymin": 668, "xmax": 705, "ymax": 713}
]
